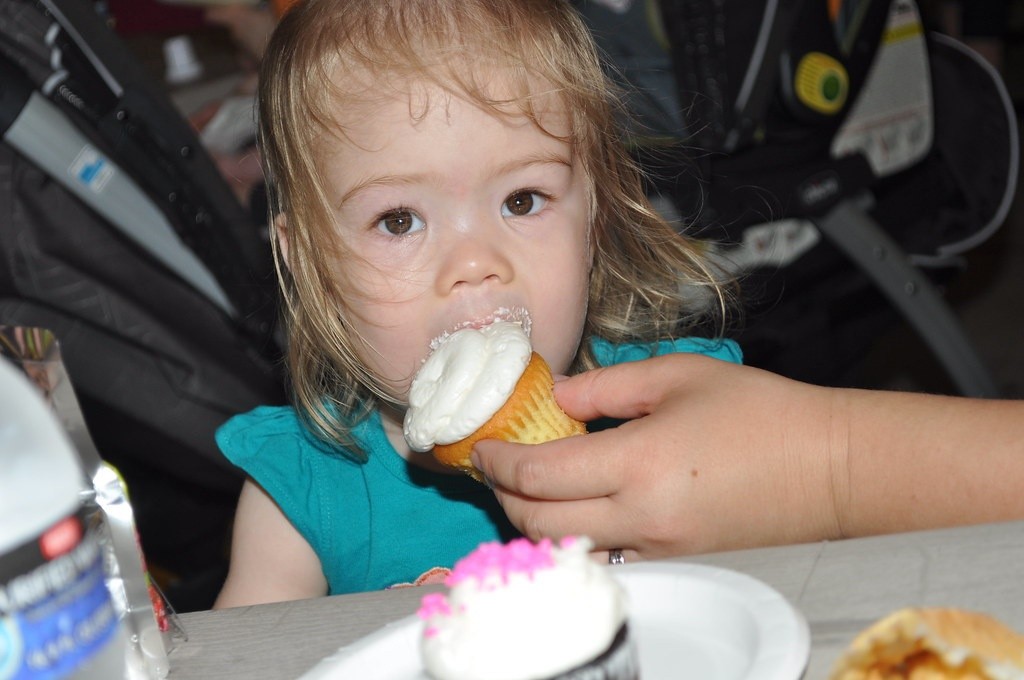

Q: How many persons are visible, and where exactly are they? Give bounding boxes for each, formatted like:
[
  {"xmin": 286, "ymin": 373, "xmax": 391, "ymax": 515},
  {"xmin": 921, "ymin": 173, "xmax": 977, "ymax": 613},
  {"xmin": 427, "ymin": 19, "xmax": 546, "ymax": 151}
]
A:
[
  {"xmin": 471, "ymin": 351, "xmax": 1024, "ymax": 565},
  {"xmin": 214, "ymin": 0, "xmax": 743, "ymax": 609}
]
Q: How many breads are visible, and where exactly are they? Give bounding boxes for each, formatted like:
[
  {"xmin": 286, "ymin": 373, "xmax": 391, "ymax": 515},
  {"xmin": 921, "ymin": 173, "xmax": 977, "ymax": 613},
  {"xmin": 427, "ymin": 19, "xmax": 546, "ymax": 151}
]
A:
[{"xmin": 824, "ymin": 606, "xmax": 1024, "ymax": 679}]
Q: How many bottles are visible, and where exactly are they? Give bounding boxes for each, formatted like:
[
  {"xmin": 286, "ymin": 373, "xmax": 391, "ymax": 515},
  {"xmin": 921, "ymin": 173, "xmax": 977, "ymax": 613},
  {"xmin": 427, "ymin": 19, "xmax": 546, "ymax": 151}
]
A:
[
  {"xmin": 13, "ymin": 326, "xmax": 170, "ymax": 680},
  {"xmin": 0, "ymin": 355, "xmax": 129, "ymax": 680}
]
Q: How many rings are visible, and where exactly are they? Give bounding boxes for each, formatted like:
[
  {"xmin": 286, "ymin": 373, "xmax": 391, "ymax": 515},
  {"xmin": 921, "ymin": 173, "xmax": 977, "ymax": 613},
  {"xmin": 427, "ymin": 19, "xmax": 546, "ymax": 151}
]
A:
[{"xmin": 609, "ymin": 549, "xmax": 624, "ymax": 565}]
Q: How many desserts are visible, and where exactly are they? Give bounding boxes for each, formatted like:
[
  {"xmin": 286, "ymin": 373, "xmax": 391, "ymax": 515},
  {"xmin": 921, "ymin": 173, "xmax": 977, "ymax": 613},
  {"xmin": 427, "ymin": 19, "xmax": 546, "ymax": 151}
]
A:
[
  {"xmin": 416, "ymin": 534, "xmax": 639, "ymax": 680},
  {"xmin": 403, "ymin": 322, "xmax": 590, "ymax": 486}
]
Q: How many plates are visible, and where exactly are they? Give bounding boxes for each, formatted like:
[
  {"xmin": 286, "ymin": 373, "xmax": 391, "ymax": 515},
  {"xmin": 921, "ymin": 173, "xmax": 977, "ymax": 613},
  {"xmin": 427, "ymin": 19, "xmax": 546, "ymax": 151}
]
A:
[{"xmin": 298, "ymin": 561, "xmax": 809, "ymax": 680}]
